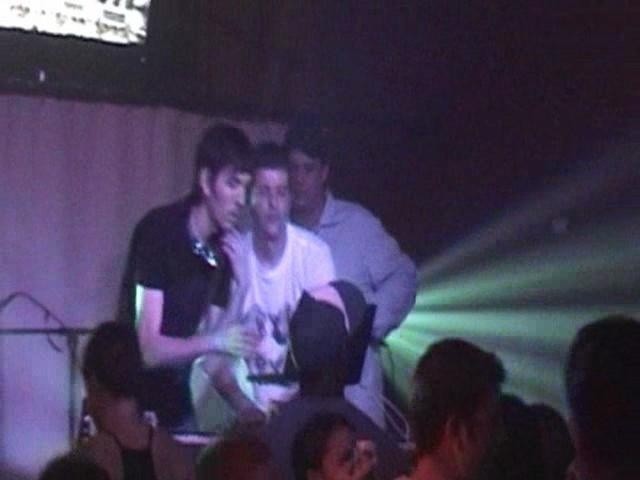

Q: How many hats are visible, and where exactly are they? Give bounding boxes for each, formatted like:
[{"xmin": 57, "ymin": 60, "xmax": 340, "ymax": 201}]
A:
[{"xmin": 289, "ymin": 279, "xmax": 378, "ymax": 368}]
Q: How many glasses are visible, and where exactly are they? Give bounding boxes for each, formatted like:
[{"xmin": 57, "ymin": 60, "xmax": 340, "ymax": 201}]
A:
[{"xmin": 190, "ymin": 238, "xmax": 222, "ymax": 269}]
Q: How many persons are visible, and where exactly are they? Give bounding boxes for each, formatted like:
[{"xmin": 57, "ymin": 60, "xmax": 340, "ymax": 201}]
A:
[
  {"xmin": 260, "ymin": 280, "xmax": 386, "ymax": 480},
  {"xmin": 278, "ymin": 124, "xmax": 420, "ymax": 435},
  {"xmin": 38, "ymin": 457, "xmax": 111, "ymax": 480},
  {"xmin": 477, "ymin": 318, "xmax": 639, "ymax": 480},
  {"xmin": 74, "ymin": 321, "xmax": 195, "ymax": 480},
  {"xmin": 390, "ymin": 338, "xmax": 506, "ymax": 480},
  {"xmin": 292, "ymin": 412, "xmax": 378, "ymax": 480},
  {"xmin": 197, "ymin": 434, "xmax": 284, "ymax": 480},
  {"xmin": 194, "ymin": 139, "xmax": 341, "ymax": 432},
  {"xmin": 114, "ymin": 121, "xmax": 269, "ymax": 466}
]
